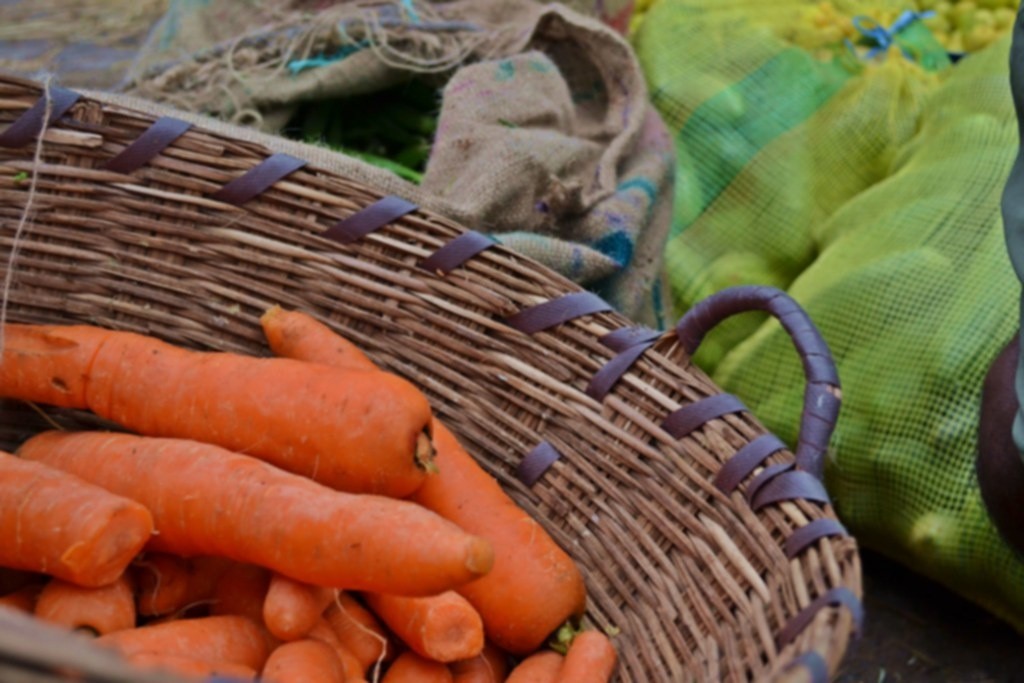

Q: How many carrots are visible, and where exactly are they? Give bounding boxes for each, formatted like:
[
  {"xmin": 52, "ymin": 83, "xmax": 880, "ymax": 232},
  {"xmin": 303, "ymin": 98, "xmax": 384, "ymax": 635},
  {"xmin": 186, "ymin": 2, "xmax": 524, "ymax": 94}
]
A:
[{"xmin": 0, "ymin": 306, "xmax": 618, "ymax": 683}]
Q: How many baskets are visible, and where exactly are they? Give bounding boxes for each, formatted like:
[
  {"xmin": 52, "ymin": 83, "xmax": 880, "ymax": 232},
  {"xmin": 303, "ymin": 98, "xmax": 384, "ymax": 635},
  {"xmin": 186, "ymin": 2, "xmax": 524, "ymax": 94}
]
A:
[{"xmin": 0, "ymin": 65, "xmax": 865, "ymax": 683}]
